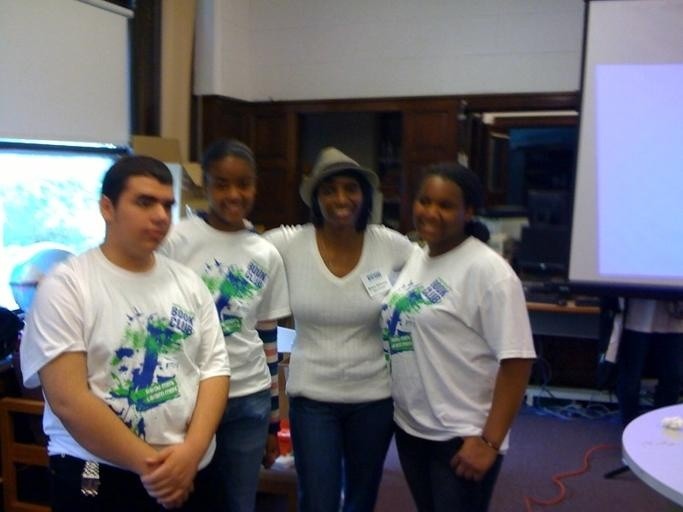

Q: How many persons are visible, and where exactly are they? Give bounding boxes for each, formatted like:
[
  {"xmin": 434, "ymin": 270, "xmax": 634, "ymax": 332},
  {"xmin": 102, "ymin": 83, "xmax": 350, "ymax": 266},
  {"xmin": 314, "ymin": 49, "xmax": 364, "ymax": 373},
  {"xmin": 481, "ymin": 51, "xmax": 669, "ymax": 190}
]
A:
[
  {"xmin": 598, "ymin": 291, "xmax": 683, "ymax": 424},
  {"xmin": 254, "ymin": 144, "xmax": 420, "ymax": 511},
  {"xmin": 158, "ymin": 136, "xmax": 295, "ymax": 510},
  {"xmin": 19, "ymin": 156, "xmax": 233, "ymax": 511},
  {"xmin": 381, "ymin": 160, "xmax": 538, "ymax": 511}
]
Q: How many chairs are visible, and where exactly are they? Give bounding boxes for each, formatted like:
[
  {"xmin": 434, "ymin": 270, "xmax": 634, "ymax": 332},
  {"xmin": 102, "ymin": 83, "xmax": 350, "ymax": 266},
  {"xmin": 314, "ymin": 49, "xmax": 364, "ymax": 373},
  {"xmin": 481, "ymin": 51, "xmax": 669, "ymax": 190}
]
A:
[{"xmin": 0, "ymin": 394, "xmax": 54, "ymax": 511}]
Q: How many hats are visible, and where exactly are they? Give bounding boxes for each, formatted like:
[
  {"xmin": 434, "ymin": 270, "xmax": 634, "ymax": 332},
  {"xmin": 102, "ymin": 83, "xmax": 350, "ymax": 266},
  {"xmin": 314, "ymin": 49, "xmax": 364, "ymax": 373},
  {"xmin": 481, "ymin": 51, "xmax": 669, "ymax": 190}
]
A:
[{"xmin": 301, "ymin": 147, "xmax": 380, "ymax": 209}]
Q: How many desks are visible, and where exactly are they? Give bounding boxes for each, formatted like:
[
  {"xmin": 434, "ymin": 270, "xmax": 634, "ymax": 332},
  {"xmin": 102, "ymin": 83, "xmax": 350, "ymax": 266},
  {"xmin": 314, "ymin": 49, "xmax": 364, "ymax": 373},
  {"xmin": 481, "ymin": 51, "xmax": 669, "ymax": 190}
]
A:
[{"xmin": 618, "ymin": 399, "xmax": 682, "ymax": 509}]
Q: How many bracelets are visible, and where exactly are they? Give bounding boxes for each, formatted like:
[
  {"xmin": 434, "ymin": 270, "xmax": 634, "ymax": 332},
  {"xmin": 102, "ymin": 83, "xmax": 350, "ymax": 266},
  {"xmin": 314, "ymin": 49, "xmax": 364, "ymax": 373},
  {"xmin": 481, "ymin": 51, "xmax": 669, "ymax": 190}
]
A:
[{"xmin": 480, "ymin": 435, "xmax": 500, "ymax": 452}]
